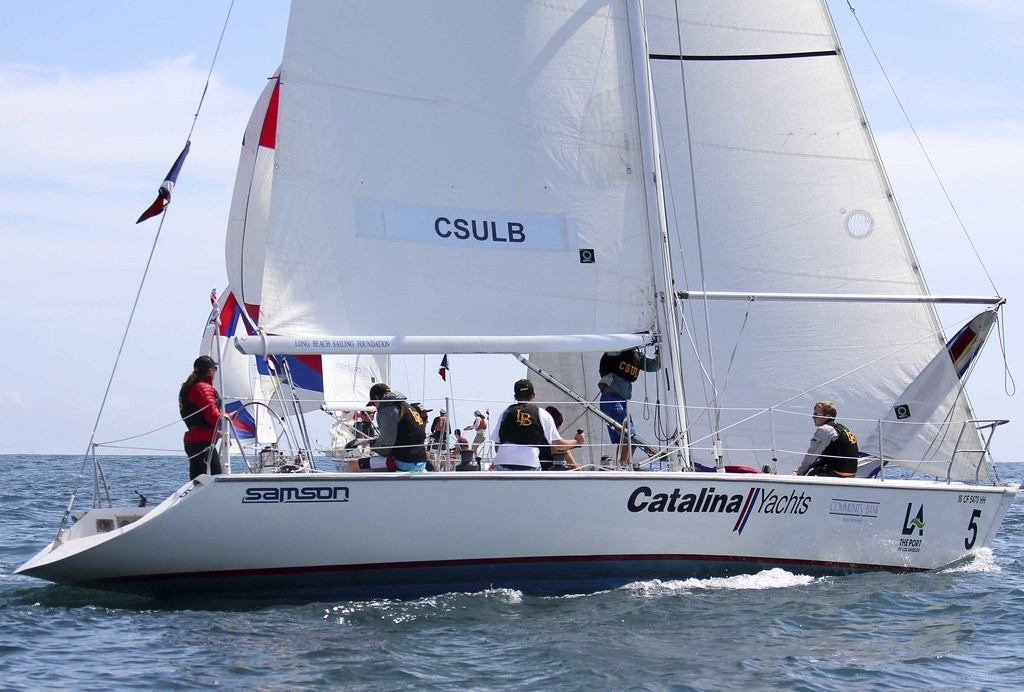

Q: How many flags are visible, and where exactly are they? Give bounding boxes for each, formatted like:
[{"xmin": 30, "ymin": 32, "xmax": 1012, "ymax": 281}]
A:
[
  {"xmin": 438, "ymin": 354, "xmax": 449, "ymax": 382},
  {"xmin": 211, "ymin": 292, "xmax": 221, "ymax": 326},
  {"xmin": 135, "ymin": 141, "xmax": 191, "ymax": 225}
]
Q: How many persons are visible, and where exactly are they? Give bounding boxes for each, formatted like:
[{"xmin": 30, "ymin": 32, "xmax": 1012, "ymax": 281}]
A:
[
  {"xmin": 597, "ymin": 343, "xmax": 660, "ymax": 470},
  {"xmin": 490, "ymin": 378, "xmax": 585, "ymax": 472},
  {"xmin": 178, "ymin": 355, "xmax": 237, "ymax": 481},
  {"xmin": 429, "ymin": 407, "xmax": 487, "ymax": 470},
  {"xmin": 796, "ymin": 401, "xmax": 858, "ymax": 478},
  {"xmin": 345, "ymin": 383, "xmax": 437, "ymax": 472}
]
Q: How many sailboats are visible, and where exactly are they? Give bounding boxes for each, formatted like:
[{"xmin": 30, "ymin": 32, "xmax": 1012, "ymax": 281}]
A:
[{"xmin": 13, "ymin": 0, "xmax": 1021, "ymax": 602}]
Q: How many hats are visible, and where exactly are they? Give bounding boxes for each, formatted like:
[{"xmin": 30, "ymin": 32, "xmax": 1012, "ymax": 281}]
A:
[
  {"xmin": 475, "ymin": 409, "xmax": 481, "ymax": 415},
  {"xmin": 410, "ymin": 403, "xmax": 433, "ymax": 413},
  {"xmin": 514, "ymin": 379, "xmax": 534, "ymax": 397},
  {"xmin": 440, "ymin": 407, "xmax": 446, "ymax": 414},
  {"xmin": 194, "ymin": 356, "xmax": 218, "ymax": 371},
  {"xmin": 366, "ymin": 384, "xmax": 387, "ymax": 406}
]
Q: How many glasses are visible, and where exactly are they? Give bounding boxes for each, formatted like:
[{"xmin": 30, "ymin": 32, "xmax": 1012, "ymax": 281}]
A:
[
  {"xmin": 212, "ymin": 366, "xmax": 217, "ymax": 372},
  {"xmin": 814, "ymin": 412, "xmax": 825, "ymax": 418}
]
